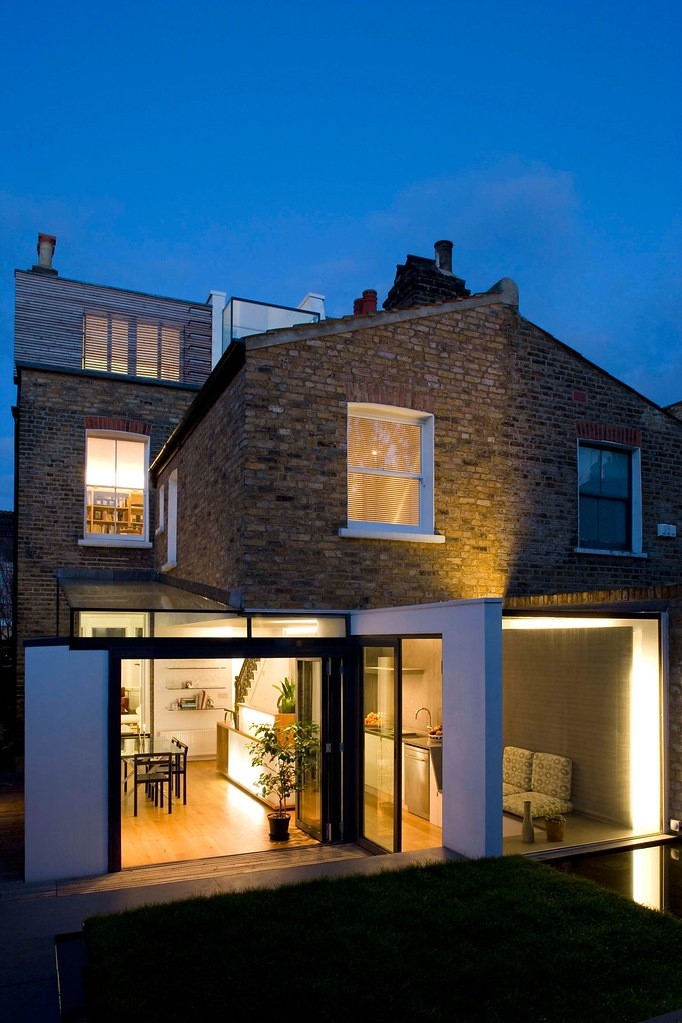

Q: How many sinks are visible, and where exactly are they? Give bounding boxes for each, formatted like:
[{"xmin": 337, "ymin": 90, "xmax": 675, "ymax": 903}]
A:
[
  {"xmin": 389, "ymin": 731, "xmax": 427, "ymax": 738},
  {"xmin": 368, "ymin": 725, "xmax": 394, "ymax": 739}
]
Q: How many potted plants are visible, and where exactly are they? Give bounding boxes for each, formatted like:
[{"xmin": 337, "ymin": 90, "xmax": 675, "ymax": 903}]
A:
[
  {"xmin": 273, "ymin": 677, "xmax": 298, "ymax": 748},
  {"xmin": 244, "ymin": 721, "xmax": 319, "ymax": 841},
  {"xmin": 544, "ymin": 810, "xmax": 566, "ymax": 842}
]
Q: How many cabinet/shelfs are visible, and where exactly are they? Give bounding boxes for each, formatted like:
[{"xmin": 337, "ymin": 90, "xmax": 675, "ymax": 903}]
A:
[
  {"xmin": 364, "ymin": 729, "xmax": 404, "ymax": 808},
  {"xmin": 86, "ymin": 489, "xmax": 144, "ymax": 536},
  {"xmin": 163, "ymin": 667, "xmax": 226, "ymax": 710},
  {"xmin": 216, "ymin": 703, "xmax": 296, "ymax": 810}
]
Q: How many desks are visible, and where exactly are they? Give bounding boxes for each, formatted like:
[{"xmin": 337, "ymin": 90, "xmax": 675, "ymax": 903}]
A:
[{"xmin": 121, "ymin": 736, "xmax": 184, "ymax": 794}]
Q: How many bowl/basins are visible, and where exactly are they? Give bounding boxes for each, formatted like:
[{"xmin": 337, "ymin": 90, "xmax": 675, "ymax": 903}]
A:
[{"xmin": 430, "ymin": 735, "xmax": 443, "ymax": 742}]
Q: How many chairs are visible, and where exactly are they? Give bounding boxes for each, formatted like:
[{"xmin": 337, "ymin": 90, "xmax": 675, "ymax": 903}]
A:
[{"xmin": 134, "ymin": 737, "xmax": 188, "ymax": 817}]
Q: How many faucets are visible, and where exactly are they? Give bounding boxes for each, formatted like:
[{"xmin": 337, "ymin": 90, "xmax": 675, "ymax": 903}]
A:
[
  {"xmin": 436, "ymin": 707, "xmax": 443, "ymax": 724},
  {"xmin": 415, "ymin": 707, "xmax": 432, "ymax": 729}
]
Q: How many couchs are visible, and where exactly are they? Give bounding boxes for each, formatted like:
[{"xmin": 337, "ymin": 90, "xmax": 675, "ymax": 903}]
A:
[{"xmin": 502, "ymin": 745, "xmax": 573, "ymax": 819}]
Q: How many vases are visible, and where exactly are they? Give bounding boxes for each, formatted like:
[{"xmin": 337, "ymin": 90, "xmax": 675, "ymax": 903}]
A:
[{"xmin": 521, "ymin": 800, "xmax": 535, "ymax": 843}]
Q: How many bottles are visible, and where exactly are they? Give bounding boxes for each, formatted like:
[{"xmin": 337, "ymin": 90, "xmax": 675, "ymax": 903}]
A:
[{"xmin": 522, "ymin": 801, "xmax": 534, "ymax": 842}]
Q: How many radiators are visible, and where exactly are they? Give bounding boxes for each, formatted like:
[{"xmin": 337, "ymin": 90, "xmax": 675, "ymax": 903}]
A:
[{"xmin": 160, "ymin": 730, "xmax": 216, "ymax": 756}]
